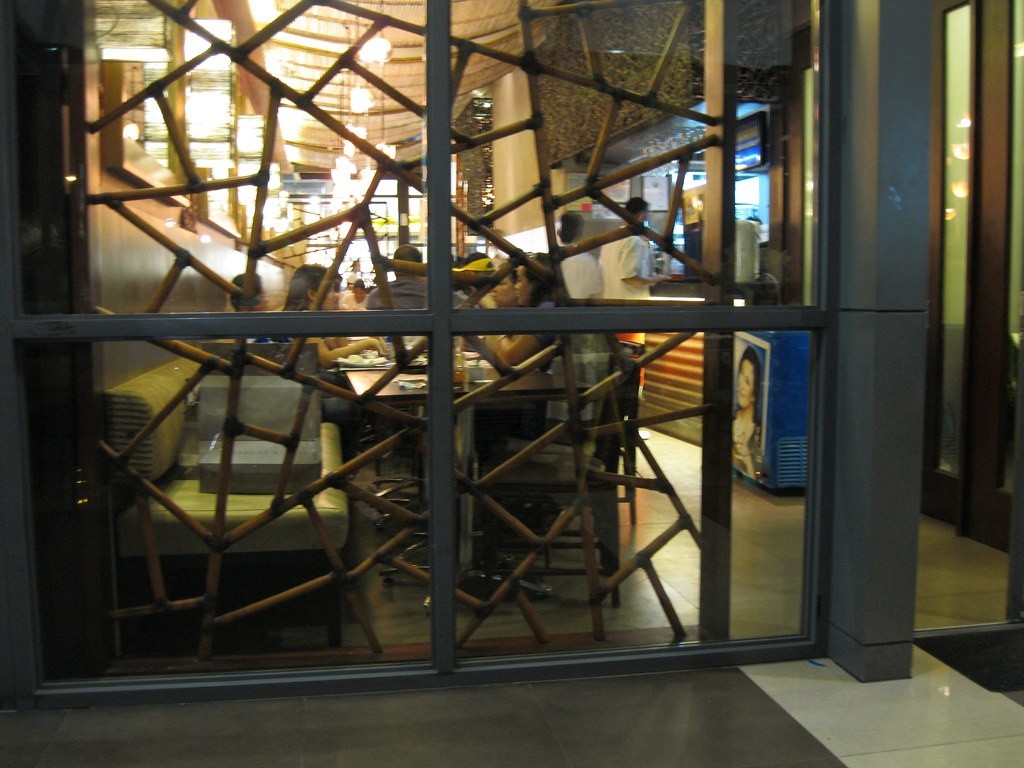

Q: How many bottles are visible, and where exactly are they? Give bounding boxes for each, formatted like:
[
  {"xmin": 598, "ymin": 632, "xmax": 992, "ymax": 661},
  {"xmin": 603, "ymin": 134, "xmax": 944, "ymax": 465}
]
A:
[{"xmin": 453, "ymin": 336, "xmax": 465, "ymax": 384}]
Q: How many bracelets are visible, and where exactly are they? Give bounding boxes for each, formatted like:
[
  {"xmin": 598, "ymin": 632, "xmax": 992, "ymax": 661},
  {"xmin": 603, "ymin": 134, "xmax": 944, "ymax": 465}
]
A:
[{"xmin": 498, "ymin": 335, "xmax": 506, "ymax": 342}]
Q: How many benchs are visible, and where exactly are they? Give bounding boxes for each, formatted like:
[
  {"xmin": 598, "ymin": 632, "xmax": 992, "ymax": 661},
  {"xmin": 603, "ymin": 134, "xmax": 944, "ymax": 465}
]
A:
[{"xmin": 107, "ymin": 351, "xmax": 351, "ymax": 647}]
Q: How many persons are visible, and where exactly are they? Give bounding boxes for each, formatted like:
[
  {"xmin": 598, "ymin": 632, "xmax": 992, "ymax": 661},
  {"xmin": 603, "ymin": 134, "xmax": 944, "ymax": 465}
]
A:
[
  {"xmin": 368, "ymin": 246, "xmax": 463, "ymax": 310},
  {"xmin": 454, "ymin": 252, "xmax": 589, "ymax": 505},
  {"xmin": 333, "ymin": 258, "xmax": 375, "ymax": 316},
  {"xmin": 230, "ymin": 273, "xmax": 272, "ymax": 344},
  {"xmin": 253, "ymin": 266, "xmax": 393, "ymax": 481},
  {"xmin": 597, "ymin": 198, "xmax": 671, "ymax": 440},
  {"xmin": 555, "ymin": 210, "xmax": 630, "ymax": 444},
  {"xmin": 731, "ymin": 345, "xmax": 764, "ymax": 480}
]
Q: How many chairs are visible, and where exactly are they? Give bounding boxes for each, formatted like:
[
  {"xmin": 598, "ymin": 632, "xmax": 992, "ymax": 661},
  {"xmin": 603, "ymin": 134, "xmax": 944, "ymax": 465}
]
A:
[{"xmin": 450, "ymin": 338, "xmax": 645, "ymax": 609}]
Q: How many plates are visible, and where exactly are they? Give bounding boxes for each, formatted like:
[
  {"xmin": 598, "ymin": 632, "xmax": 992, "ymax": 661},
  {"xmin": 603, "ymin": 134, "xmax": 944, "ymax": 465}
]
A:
[{"xmin": 463, "ymin": 352, "xmax": 481, "ymax": 360}]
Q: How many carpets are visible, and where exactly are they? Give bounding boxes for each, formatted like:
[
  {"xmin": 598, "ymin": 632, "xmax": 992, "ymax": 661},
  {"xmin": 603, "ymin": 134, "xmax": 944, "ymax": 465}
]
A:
[{"xmin": 913, "ymin": 627, "xmax": 1024, "ymax": 692}]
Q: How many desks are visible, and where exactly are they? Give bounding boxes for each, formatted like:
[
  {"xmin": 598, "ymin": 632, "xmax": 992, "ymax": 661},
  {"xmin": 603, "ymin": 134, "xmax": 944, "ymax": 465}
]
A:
[{"xmin": 342, "ymin": 352, "xmax": 600, "ymax": 598}]
